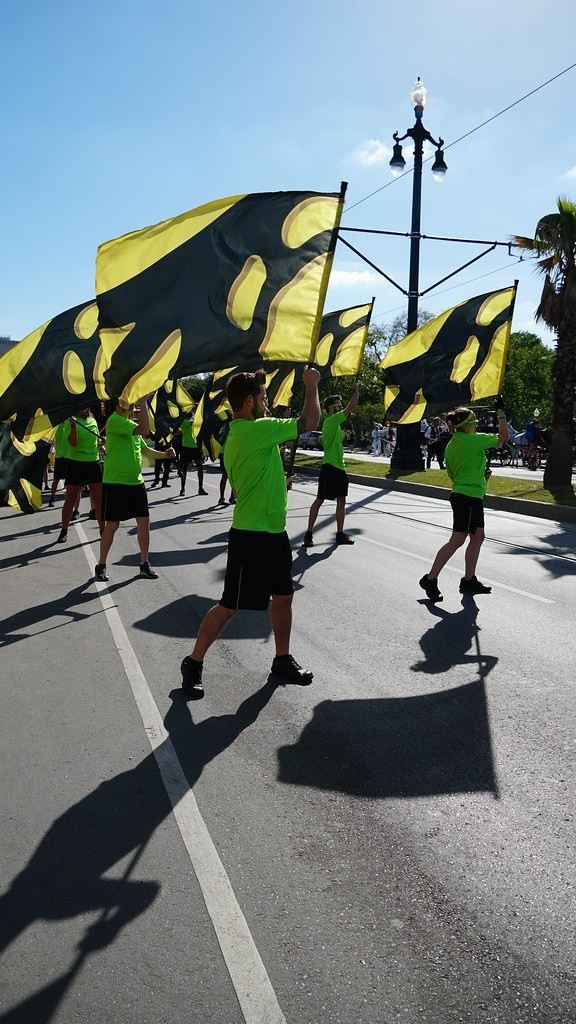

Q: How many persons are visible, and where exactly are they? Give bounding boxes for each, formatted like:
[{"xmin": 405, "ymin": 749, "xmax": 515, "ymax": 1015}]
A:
[
  {"xmin": 345, "ymin": 411, "xmax": 452, "ymax": 469},
  {"xmin": 48, "ymin": 406, "xmax": 172, "ymax": 542},
  {"xmin": 180, "ymin": 371, "xmax": 320, "ymax": 700},
  {"xmin": 303, "ymin": 383, "xmax": 360, "ymax": 548},
  {"xmin": 179, "ymin": 410, "xmax": 240, "ymax": 507},
  {"xmin": 94, "ymin": 398, "xmax": 177, "ymax": 579},
  {"xmin": 419, "ymin": 405, "xmax": 509, "ymax": 601}
]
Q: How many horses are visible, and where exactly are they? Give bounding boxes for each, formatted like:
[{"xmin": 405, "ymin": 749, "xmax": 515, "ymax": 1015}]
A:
[{"xmin": 506, "ymin": 423, "xmax": 527, "ymax": 469}]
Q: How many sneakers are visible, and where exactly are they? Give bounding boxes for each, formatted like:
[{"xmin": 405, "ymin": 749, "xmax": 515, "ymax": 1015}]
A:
[
  {"xmin": 303, "ymin": 535, "xmax": 316, "ymax": 547},
  {"xmin": 419, "ymin": 574, "xmax": 444, "ymax": 601},
  {"xmin": 271, "ymin": 655, "xmax": 314, "ymax": 681},
  {"xmin": 337, "ymin": 533, "xmax": 355, "ymax": 545},
  {"xmin": 139, "ymin": 562, "xmax": 158, "ymax": 579},
  {"xmin": 95, "ymin": 563, "xmax": 110, "ymax": 581},
  {"xmin": 181, "ymin": 655, "xmax": 204, "ymax": 697},
  {"xmin": 459, "ymin": 575, "xmax": 492, "ymax": 592}
]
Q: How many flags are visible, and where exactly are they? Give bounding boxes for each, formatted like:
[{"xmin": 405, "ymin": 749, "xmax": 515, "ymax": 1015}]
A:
[
  {"xmin": 378, "ymin": 287, "xmax": 517, "ymax": 424},
  {"xmin": 96, "ymin": 191, "xmax": 347, "ymax": 399},
  {"xmin": 1, "ymin": 302, "xmax": 306, "ymax": 516},
  {"xmin": 308, "ymin": 304, "xmax": 373, "ymax": 375},
  {"xmin": 191, "ymin": 367, "xmax": 300, "ymax": 463}
]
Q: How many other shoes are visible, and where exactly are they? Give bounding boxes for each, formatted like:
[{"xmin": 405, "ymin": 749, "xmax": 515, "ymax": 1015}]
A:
[
  {"xmin": 88, "ymin": 509, "xmax": 97, "ymax": 520},
  {"xmin": 218, "ymin": 498, "xmax": 231, "ymax": 506},
  {"xmin": 71, "ymin": 510, "xmax": 80, "ymax": 520},
  {"xmin": 162, "ymin": 483, "xmax": 171, "ymax": 487},
  {"xmin": 229, "ymin": 497, "xmax": 237, "ymax": 503},
  {"xmin": 151, "ymin": 480, "xmax": 159, "ymax": 487},
  {"xmin": 49, "ymin": 499, "xmax": 55, "ymax": 507},
  {"xmin": 180, "ymin": 489, "xmax": 186, "ymax": 496},
  {"xmin": 199, "ymin": 488, "xmax": 208, "ymax": 495},
  {"xmin": 45, "ymin": 486, "xmax": 52, "ymax": 490},
  {"xmin": 58, "ymin": 530, "xmax": 67, "ymax": 543}
]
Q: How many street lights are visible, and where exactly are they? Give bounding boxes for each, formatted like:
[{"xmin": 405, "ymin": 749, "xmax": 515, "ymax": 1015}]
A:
[{"xmin": 388, "ymin": 77, "xmax": 449, "ymax": 472}]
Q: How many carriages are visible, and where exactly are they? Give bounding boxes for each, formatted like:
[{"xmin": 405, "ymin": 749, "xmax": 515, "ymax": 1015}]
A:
[{"xmin": 467, "ymin": 405, "xmax": 576, "ymax": 471}]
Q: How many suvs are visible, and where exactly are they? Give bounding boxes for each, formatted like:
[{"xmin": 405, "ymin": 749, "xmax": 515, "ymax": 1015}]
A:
[{"xmin": 297, "ymin": 431, "xmax": 322, "ymax": 450}]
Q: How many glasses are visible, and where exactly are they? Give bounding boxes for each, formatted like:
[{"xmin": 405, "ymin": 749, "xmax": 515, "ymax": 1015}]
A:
[
  {"xmin": 468, "ymin": 420, "xmax": 479, "ymax": 427},
  {"xmin": 328, "ymin": 404, "xmax": 342, "ymax": 408}
]
